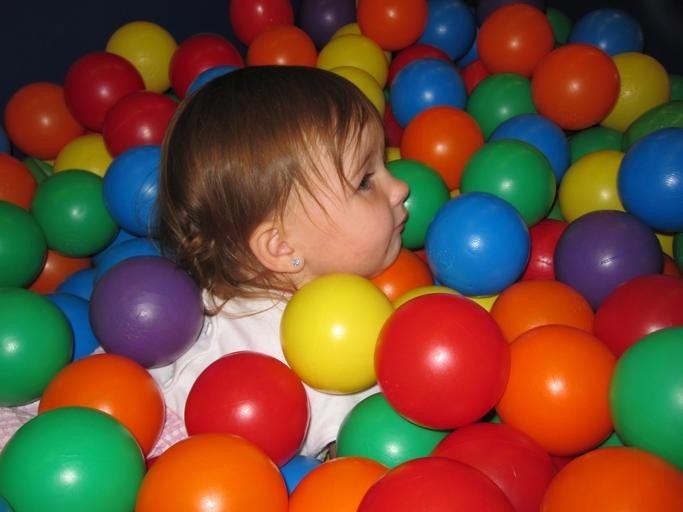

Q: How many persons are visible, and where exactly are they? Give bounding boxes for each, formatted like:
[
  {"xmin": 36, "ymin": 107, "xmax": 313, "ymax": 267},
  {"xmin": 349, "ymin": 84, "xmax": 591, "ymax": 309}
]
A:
[{"xmin": 2, "ymin": 64, "xmax": 411, "ymax": 460}]
